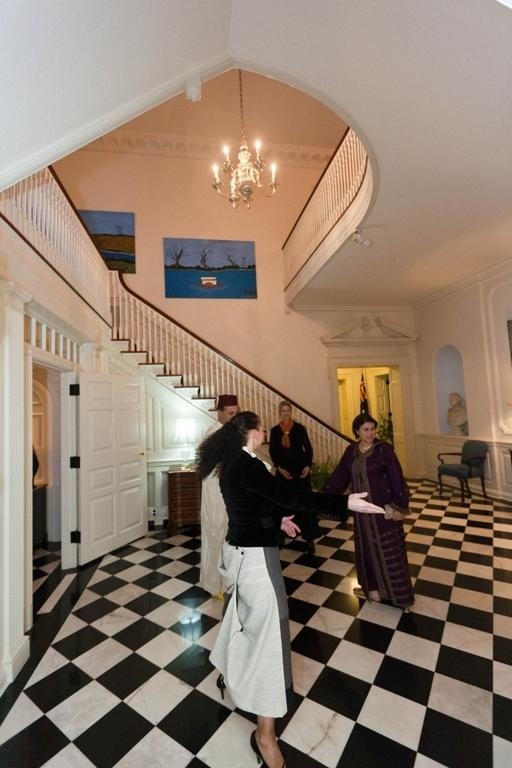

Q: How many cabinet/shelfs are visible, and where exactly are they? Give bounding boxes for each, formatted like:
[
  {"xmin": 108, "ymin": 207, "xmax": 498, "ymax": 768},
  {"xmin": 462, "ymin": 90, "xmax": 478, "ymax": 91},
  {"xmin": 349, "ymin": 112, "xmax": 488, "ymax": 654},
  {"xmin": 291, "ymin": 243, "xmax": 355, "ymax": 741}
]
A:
[
  {"xmin": 166, "ymin": 466, "xmax": 202, "ymax": 530},
  {"xmin": 34, "ymin": 483, "xmax": 49, "ymax": 553}
]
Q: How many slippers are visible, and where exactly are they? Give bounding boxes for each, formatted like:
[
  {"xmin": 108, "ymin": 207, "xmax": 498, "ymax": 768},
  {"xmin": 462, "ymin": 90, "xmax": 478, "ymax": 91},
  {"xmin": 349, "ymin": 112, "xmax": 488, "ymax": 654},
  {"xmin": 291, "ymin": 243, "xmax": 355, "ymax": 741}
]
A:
[{"xmin": 354, "ymin": 589, "xmax": 381, "ymax": 601}]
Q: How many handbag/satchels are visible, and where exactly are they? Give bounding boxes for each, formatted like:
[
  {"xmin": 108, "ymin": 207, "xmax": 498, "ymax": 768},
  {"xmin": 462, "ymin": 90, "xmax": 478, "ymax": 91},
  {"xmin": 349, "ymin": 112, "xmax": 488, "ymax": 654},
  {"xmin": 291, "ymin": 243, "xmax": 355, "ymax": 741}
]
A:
[{"xmin": 384, "ymin": 503, "xmax": 412, "ymax": 520}]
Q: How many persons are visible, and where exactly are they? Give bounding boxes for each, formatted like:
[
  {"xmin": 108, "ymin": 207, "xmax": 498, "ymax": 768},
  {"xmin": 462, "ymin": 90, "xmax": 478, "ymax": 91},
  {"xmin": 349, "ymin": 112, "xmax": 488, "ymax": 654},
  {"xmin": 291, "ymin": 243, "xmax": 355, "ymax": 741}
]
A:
[
  {"xmin": 320, "ymin": 412, "xmax": 416, "ymax": 615},
  {"xmin": 271, "ymin": 401, "xmax": 314, "ymax": 556},
  {"xmin": 446, "ymin": 392, "xmax": 467, "ymax": 436},
  {"xmin": 196, "ymin": 413, "xmax": 388, "ymax": 767},
  {"xmin": 198, "ymin": 393, "xmax": 278, "ymax": 596}
]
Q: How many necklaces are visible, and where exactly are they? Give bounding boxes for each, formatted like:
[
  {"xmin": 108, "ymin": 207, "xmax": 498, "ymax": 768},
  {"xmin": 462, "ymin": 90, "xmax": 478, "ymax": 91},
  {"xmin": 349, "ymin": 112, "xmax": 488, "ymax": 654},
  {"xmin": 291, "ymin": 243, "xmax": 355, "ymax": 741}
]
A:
[
  {"xmin": 280, "ymin": 423, "xmax": 291, "ymax": 434},
  {"xmin": 359, "ymin": 441, "xmax": 374, "ymax": 450}
]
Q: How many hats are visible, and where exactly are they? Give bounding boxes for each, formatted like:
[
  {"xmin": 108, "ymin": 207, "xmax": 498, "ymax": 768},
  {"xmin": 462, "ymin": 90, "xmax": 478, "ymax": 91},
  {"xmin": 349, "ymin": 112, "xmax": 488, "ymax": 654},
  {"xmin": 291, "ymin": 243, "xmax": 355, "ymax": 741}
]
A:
[{"xmin": 218, "ymin": 395, "xmax": 237, "ymax": 406}]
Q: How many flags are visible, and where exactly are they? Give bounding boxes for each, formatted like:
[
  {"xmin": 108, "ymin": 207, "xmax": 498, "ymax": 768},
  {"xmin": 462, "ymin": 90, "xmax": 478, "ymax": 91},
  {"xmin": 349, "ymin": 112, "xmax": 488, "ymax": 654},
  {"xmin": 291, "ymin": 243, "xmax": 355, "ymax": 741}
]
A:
[{"xmin": 360, "ymin": 371, "xmax": 369, "ymax": 411}]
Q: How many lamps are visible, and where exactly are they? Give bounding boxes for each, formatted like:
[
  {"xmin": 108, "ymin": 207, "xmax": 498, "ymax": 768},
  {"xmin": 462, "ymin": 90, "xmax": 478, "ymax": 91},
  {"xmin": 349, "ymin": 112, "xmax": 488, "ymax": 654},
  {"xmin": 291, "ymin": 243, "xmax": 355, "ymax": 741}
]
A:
[{"xmin": 207, "ymin": 73, "xmax": 282, "ymax": 211}]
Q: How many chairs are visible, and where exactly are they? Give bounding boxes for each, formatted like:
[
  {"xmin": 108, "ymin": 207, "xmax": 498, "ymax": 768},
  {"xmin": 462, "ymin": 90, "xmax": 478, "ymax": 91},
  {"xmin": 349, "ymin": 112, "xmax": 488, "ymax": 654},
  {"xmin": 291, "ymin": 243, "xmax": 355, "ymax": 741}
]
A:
[{"xmin": 437, "ymin": 440, "xmax": 489, "ymax": 501}]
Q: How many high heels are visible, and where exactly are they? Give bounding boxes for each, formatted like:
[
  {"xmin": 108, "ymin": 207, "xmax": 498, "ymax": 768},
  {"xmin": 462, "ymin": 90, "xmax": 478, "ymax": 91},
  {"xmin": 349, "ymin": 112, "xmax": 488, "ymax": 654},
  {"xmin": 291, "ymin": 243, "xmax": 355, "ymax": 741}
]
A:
[
  {"xmin": 217, "ymin": 674, "xmax": 226, "ymax": 698},
  {"xmin": 251, "ymin": 730, "xmax": 289, "ymax": 768}
]
